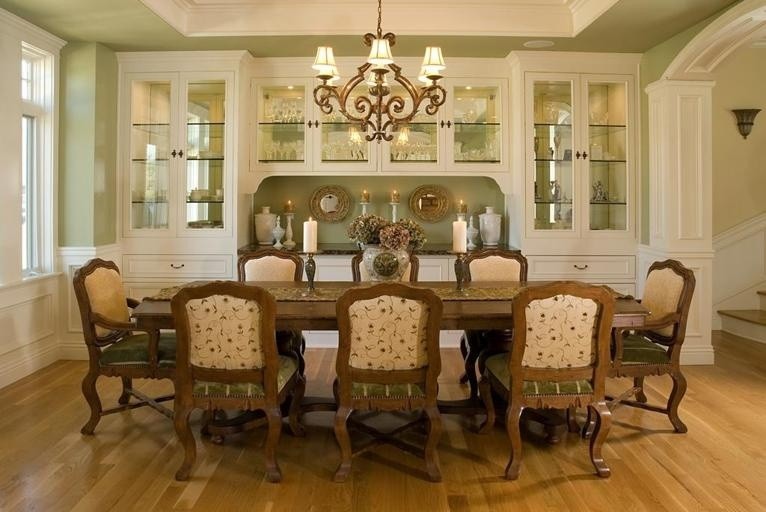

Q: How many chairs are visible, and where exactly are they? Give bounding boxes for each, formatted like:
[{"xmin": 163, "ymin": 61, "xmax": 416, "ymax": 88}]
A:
[
  {"xmin": 236, "ymin": 250, "xmax": 307, "ymax": 379},
  {"xmin": 607, "ymin": 258, "xmax": 701, "ymax": 433},
  {"xmin": 169, "ymin": 280, "xmax": 301, "ymax": 483},
  {"xmin": 479, "ymin": 281, "xmax": 621, "ymax": 482},
  {"xmin": 454, "ymin": 245, "xmax": 529, "ymax": 406},
  {"xmin": 70, "ymin": 257, "xmax": 179, "ymax": 437},
  {"xmin": 331, "ymin": 280, "xmax": 447, "ymax": 484}
]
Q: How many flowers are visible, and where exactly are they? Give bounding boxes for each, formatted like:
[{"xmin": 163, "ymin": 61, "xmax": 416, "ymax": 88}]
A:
[{"xmin": 347, "ymin": 213, "xmax": 424, "ymax": 248}]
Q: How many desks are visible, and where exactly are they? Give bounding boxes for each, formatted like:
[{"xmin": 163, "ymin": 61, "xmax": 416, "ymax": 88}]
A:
[{"xmin": 128, "ymin": 281, "xmax": 653, "ymax": 436}]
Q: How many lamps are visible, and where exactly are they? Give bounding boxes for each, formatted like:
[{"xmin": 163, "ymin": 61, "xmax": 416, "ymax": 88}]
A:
[
  {"xmin": 731, "ymin": 108, "xmax": 762, "ymax": 138},
  {"xmin": 311, "ymin": 0, "xmax": 449, "ymax": 144}
]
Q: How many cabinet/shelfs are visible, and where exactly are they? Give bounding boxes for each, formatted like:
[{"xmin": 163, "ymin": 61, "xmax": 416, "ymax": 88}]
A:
[
  {"xmin": 504, "ymin": 50, "xmax": 645, "ymax": 300},
  {"xmin": 116, "ymin": 52, "xmax": 254, "ymax": 334},
  {"xmin": 245, "ymin": 53, "xmax": 516, "ymax": 199}
]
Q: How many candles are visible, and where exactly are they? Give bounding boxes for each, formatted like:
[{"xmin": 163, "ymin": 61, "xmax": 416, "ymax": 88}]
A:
[
  {"xmin": 360, "ymin": 189, "xmax": 370, "ymax": 202},
  {"xmin": 458, "ymin": 199, "xmax": 463, "ymax": 214},
  {"xmin": 391, "ymin": 189, "xmax": 400, "ymax": 202},
  {"xmin": 283, "ymin": 198, "xmax": 295, "ymax": 213},
  {"xmin": 469, "ymin": 214, "xmax": 474, "ymax": 227},
  {"xmin": 451, "ymin": 215, "xmax": 467, "ymax": 253},
  {"xmin": 302, "ymin": 216, "xmax": 319, "ymax": 253}
]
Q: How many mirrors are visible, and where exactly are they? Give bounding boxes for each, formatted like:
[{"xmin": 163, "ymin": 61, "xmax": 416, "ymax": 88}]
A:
[
  {"xmin": 409, "ymin": 184, "xmax": 450, "ymax": 224},
  {"xmin": 309, "ymin": 183, "xmax": 353, "ymax": 223}
]
